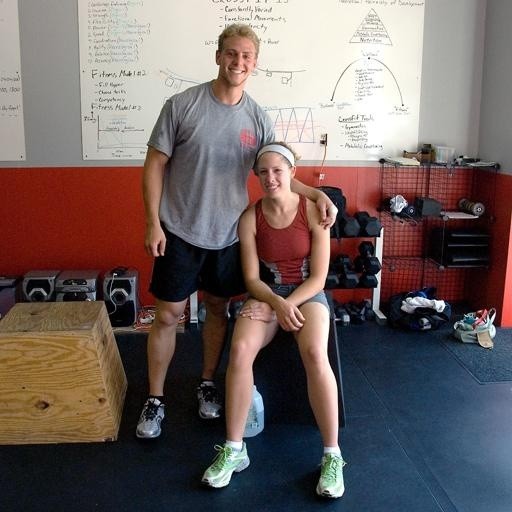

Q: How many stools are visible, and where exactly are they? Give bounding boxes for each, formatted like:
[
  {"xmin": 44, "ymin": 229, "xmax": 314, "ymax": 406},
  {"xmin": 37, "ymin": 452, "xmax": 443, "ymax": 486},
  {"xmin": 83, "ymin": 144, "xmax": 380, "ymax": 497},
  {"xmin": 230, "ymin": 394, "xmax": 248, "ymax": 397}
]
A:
[{"xmin": 232, "ymin": 297, "xmax": 347, "ymax": 427}]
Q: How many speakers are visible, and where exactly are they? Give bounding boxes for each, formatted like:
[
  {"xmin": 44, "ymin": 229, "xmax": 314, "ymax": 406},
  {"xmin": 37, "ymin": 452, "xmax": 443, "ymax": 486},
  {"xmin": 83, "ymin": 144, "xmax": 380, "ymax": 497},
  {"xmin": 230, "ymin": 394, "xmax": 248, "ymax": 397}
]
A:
[
  {"xmin": 22, "ymin": 270, "xmax": 60, "ymax": 302},
  {"xmin": 102, "ymin": 269, "xmax": 139, "ymax": 327},
  {"xmin": 56, "ymin": 269, "xmax": 100, "ymax": 302}
]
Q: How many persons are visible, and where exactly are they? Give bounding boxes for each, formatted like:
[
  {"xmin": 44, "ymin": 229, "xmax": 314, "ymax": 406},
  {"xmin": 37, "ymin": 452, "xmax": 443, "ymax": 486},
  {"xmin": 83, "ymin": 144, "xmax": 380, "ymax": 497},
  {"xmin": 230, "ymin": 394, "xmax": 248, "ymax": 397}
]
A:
[
  {"xmin": 200, "ymin": 141, "xmax": 345, "ymax": 499},
  {"xmin": 137, "ymin": 23, "xmax": 339, "ymax": 439}
]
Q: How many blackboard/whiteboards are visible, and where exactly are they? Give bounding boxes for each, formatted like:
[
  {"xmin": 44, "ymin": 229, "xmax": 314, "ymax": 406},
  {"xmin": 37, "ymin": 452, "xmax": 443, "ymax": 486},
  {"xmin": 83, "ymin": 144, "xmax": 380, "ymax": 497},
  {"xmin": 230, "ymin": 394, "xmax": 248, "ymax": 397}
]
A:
[
  {"xmin": 77, "ymin": 0, "xmax": 426, "ymax": 162},
  {"xmin": 0, "ymin": 1, "xmax": 27, "ymax": 160}
]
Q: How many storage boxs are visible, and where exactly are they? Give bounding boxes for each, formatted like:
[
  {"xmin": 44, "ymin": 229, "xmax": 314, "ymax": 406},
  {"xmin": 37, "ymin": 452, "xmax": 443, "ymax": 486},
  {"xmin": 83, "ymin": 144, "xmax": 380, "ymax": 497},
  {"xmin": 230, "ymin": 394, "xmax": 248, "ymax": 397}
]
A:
[{"xmin": 432, "ymin": 145, "xmax": 455, "ymax": 165}]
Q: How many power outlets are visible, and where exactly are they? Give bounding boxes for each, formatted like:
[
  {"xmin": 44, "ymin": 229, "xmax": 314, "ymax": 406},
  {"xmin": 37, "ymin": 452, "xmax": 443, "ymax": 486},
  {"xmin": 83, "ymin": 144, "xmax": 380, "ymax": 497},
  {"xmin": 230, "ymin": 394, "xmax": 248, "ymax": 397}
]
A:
[{"xmin": 320, "ymin": 134, "xmax": 327, "ymax": 145}]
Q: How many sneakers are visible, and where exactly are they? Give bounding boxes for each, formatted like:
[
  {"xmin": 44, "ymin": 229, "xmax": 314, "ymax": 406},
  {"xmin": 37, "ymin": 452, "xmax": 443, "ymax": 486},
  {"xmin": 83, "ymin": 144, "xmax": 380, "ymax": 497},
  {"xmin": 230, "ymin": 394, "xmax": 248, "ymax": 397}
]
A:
[
  {"xmin": 197, "ymin": 381, "xmax": 222, "ymax": 419},
  {"xmin": 201, "ymin": 442, "xmax": 250, "ymax": 489},
  {"xmin": 136, "ymin": 398, "xmax": 166, "ymax": 438},
  {"xmin": 316, "ymin": 453, "xmax": 347, "ymax": 498}
]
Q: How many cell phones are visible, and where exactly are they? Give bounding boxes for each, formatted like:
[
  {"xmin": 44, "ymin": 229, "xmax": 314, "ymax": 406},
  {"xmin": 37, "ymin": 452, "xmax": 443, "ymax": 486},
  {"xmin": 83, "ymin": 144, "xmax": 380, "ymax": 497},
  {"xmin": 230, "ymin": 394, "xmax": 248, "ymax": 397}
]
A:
[{"xmin": 111, "ymin": 266, "xmax": 128, "ymax": 276}]
